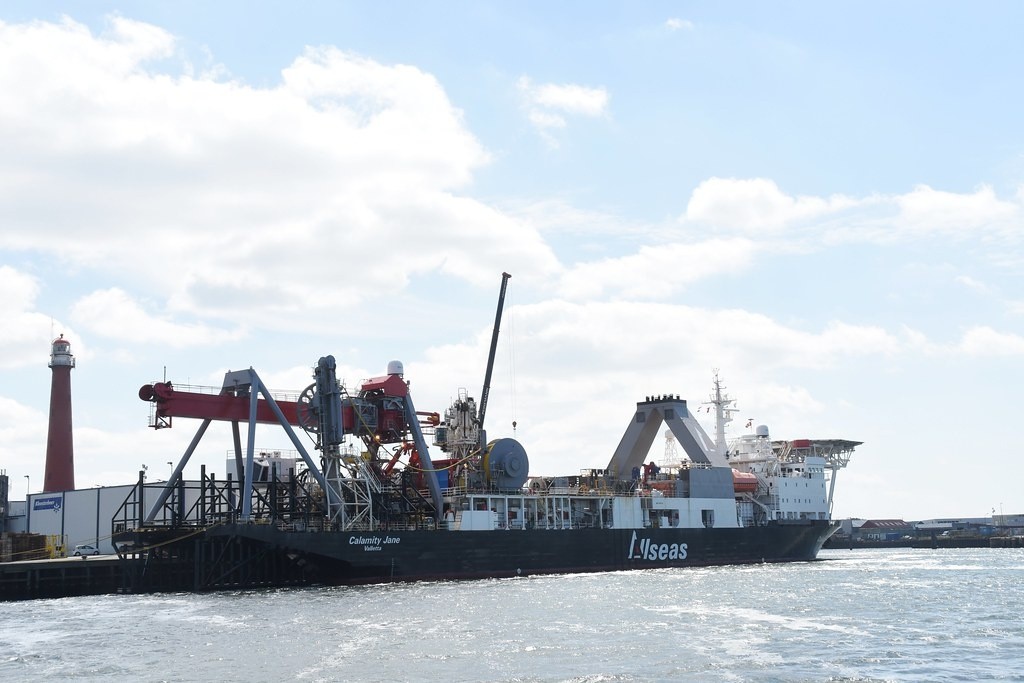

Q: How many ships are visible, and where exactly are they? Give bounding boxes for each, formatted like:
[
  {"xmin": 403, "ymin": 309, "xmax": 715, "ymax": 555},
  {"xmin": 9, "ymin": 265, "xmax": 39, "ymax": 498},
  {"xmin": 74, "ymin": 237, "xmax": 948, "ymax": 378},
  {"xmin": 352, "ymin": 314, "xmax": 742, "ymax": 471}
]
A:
[{"xmin": 110, "ymin": 270, "xmax": 867, "ymax": 600}]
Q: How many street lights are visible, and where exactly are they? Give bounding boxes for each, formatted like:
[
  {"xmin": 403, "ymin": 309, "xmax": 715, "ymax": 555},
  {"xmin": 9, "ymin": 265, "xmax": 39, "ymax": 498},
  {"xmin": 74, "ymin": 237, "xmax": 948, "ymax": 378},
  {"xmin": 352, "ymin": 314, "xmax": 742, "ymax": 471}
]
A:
[{"xmin": 21, "ymin": 473, "xmax": 32, "ymax": 532}]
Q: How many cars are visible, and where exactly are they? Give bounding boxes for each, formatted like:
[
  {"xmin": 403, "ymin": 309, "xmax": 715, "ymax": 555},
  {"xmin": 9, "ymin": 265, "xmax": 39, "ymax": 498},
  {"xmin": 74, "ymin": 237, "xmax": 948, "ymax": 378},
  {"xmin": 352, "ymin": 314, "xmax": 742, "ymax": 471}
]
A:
[{"xmin": 72, "ymin": 544, "xmax": 100, "ymax": 556}]
